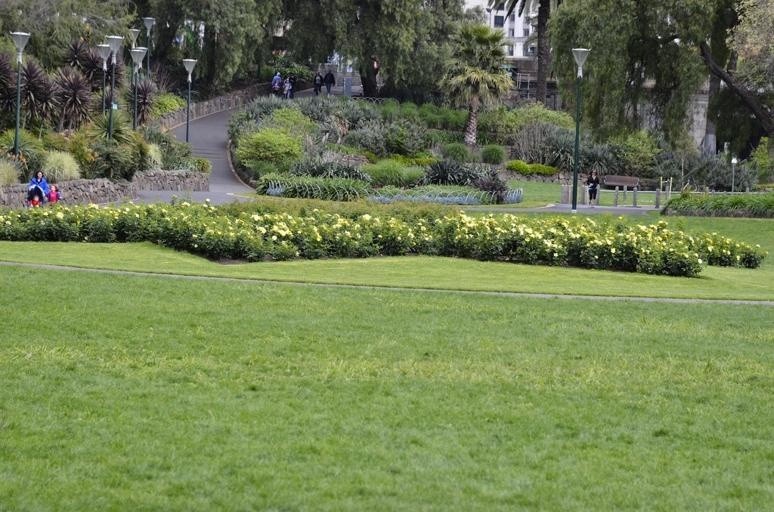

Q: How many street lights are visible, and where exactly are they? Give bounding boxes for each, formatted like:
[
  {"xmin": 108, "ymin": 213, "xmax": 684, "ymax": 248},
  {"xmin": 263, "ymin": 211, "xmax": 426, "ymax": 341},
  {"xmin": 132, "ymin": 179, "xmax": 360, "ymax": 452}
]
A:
[
  {"xmin": 7, "ymin": 28, "xmax": 34, "ymax": 160},
  {"xmin": 179, "ymin": 56, "xmax": 201, "ymax": 156},
  {"xmin": 566, "ymin": 44, "xmax": 595, "ymax": 215},
  {"xmin": 98, "ymin": 12, "xmax": 160, "ymax": 149}
]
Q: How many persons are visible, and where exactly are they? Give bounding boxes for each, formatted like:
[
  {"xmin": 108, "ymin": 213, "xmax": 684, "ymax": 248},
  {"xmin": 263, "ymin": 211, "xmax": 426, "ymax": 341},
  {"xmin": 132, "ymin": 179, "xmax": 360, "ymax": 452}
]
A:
[
  {"xmin": 283, "ymin": 78, "xmax": 292, "ymax": 99},
  {"xmin": 29, "ymin": 171, "xmax": 50, "ymax": 195},
  {"xmin": 271, "ymin": 72, "xmax": 283, "ymax": 96},
  {"xmin": 324, "ymin": 70, "xmax": 336, "ymax": 97},
  {"xmin": 28, "ymin": 194, "xmax": 42, "ymax": 208},
  {"xmin": 586, "ymin": 171, "xmax": 599, "ymax": 206},
  {"xmin": 312, "ymin": 72, "xmax": 324, "ymax": 97},
  {"xmin": 45, "ymin": 185, "xmax": 64, "ymax": 203}
]
